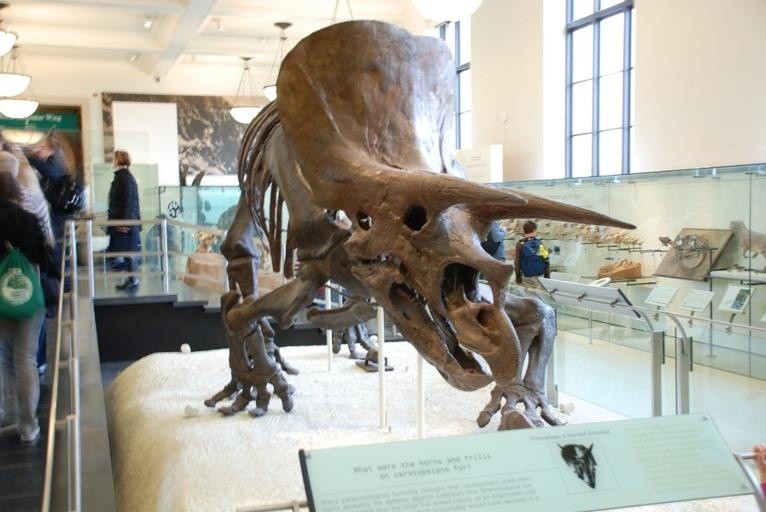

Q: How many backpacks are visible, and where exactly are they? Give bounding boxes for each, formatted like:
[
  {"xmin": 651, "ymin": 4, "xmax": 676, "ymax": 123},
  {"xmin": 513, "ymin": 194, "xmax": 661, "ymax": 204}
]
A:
[{"xmin": 519, "ymin": 239, "xmax": 545, "ymax": 277}]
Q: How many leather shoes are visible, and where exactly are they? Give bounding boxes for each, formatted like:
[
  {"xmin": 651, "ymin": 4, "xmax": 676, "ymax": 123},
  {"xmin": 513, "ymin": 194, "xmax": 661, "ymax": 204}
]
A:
[{"xmin": 114, "ymin": 277, "xmax": 139, "ymax": 290}]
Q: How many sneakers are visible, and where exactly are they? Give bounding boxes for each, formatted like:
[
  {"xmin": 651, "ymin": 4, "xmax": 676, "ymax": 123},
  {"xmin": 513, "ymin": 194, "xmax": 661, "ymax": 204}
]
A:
[
  {"xmin": 1, "ymin": 423, "xmax": 40, "ymax": 443},
  {"xmin": 37, "ymin": 361, "xmax": 46, "ymax": 374}
]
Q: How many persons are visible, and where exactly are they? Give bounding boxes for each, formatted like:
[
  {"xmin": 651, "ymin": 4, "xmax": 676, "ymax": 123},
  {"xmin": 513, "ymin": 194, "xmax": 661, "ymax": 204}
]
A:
[
  {"xmin": 25, "ymin": 135, "xmax": 85, "ymax": 321},
  {"xmin": 143, "ymin": 213, "xmax": 181, "ymax": 283},
  {"xmin": 0, "ymin": 169, "xmax": 64, "ymax": 451},
  {"xmin": 513, "ymin": 220, "xmax": 553, "ymax": 287},
  {"xmin": 0, "ymin": 148, "xmax": 58, "ymax": 378},
  {"xmin": 102, "ymin": 150, "xmax": 143, "ymax": 291},
  {"xmin": 481, "ymin": 219, "xmax": 507, "ymax": 281}
]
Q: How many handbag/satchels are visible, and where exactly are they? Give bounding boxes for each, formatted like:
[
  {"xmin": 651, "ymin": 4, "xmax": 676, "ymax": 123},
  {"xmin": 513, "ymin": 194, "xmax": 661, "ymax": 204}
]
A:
[{"xmin": 0, "ymin": 247, "xmax": 46, "ymax": 320}]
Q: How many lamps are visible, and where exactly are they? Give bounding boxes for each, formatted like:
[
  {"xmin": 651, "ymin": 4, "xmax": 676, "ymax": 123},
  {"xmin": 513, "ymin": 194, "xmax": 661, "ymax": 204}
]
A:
[
  {"xmin": 228, "ymin": 56, "xmax": 264, "ymax": 125},
  {"xmin": 0, "ymin": 84, "xmax": 39, "ymax": 119},
  {"xmin": 0, "ymin": 45, "xmax": 31, "ymax": 98},
  {"xmin": 262, "ymin": 22, "xmax": 292, "ymax": 101},
  {"xmin": 0, "ymin": 3, "xmax": 18, "ymax": 57}
]
